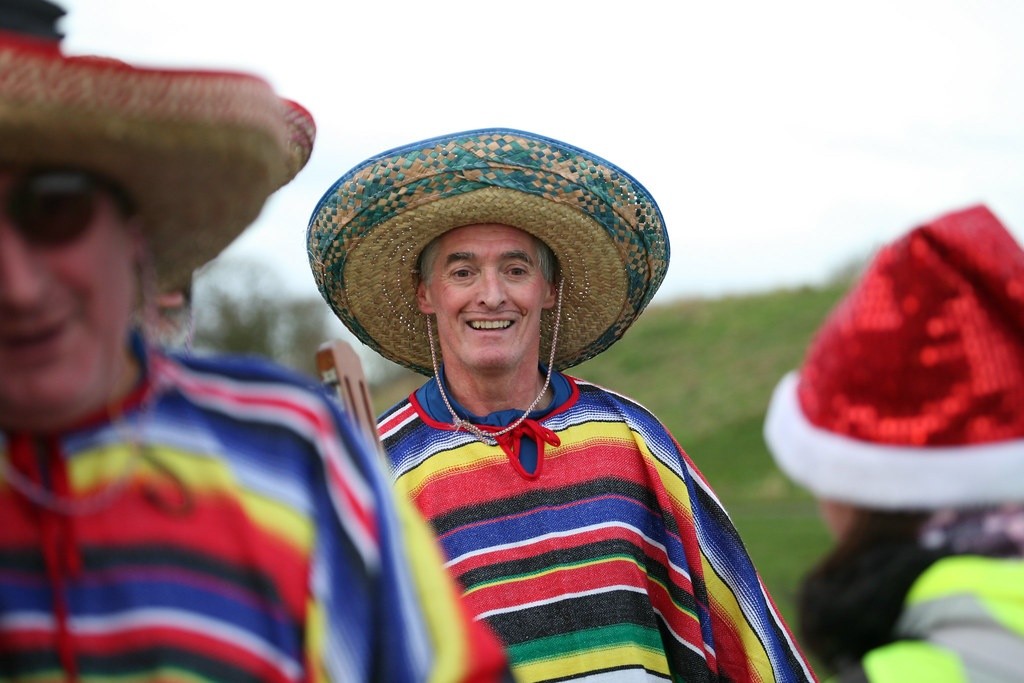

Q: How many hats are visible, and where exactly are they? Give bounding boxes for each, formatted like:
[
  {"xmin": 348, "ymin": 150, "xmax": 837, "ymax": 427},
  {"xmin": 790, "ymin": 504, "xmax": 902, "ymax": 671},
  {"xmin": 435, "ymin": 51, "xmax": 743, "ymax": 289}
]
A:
[
  {"xmin": 760, "ymin": 202, "xmax": 1024, "ymax": 512},
  {"xmin": 0, "ymin": 33, "xmax": 316, "ymax": 292},
  {"xmin": 306, "ymin": 128, "xmax": 671, "ymax": 378}
]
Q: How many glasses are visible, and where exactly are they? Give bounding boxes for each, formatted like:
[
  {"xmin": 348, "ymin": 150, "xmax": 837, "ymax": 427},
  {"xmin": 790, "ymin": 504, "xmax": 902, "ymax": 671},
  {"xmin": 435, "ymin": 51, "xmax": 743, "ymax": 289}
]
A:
[{"xmin": 0, "ymin": 162, "xmax": 134, "ymax": 244}]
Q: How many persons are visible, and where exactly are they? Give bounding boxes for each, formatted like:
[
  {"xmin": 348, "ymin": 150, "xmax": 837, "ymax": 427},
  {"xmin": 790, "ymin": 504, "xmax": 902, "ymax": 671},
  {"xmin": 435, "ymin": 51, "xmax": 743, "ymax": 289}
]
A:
[
  {"xmin": 759, "ymin": 205, "xmax": 1024, "ymax": 682},
  {"xmin": 306, "ymin": 127, "xmax": 814, "ymax": 683},
  {"xmin": 0, "ymin": 0, "xmax": 508, "ymax": 683}
]
